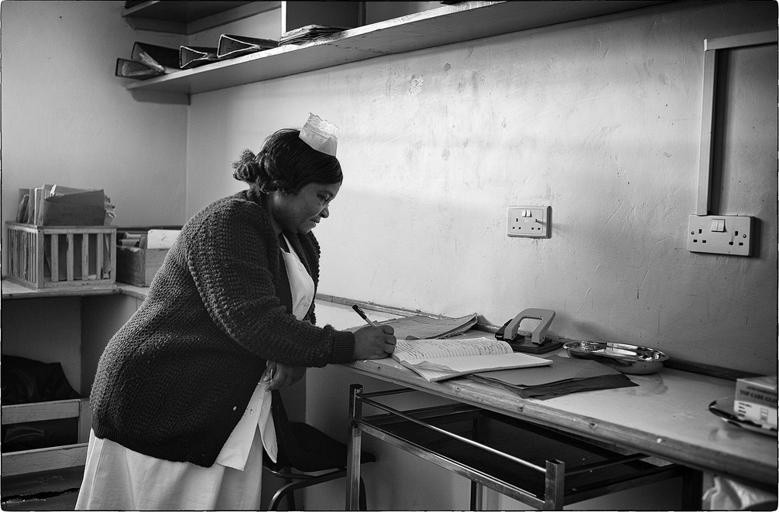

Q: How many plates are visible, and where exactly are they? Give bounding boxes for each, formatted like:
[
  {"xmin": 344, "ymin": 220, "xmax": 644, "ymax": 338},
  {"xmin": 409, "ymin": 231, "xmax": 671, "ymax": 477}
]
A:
[{"xmin": 563, "ymin": 339, "xmax": 670, "ymax": 377}]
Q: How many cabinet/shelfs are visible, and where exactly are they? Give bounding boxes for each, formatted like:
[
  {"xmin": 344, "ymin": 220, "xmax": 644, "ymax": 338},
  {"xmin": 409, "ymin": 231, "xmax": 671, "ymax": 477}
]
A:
[{"xmin": 80, "ymin": 295, "xmax": 471, "ymax": 512}]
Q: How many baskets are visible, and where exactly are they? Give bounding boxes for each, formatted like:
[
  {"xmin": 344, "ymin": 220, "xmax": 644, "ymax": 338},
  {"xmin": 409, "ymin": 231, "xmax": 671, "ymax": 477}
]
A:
[{"xmin": 6, "ymin": 218, "xmax": 117, "ymax": 290}]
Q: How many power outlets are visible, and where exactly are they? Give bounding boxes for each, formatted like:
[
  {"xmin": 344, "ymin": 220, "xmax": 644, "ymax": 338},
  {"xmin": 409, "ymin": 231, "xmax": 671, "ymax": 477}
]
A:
[
  {"xmin": 506, "ymin": 205, "xmax": 550, "ymax": 239},
  {"xmin": 686, "ymin": 214, "xmax": 756, "ymax": 256}
]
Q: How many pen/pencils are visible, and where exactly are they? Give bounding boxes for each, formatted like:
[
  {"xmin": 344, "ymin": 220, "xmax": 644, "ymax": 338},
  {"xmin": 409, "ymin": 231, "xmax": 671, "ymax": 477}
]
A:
[
  {"xmin": 352, "ymin": 304, "xmax": 376, "ymax": 327},
  {"xmin": 605, "ymin": 343, "xmax": 652, "ymax": 356}
]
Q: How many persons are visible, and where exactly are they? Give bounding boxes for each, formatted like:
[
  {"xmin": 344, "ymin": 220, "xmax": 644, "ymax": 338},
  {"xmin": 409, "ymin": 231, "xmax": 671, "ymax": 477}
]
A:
[{"xmin": 72, "ymin": 112, "xmax": 397, "ymax": 509}]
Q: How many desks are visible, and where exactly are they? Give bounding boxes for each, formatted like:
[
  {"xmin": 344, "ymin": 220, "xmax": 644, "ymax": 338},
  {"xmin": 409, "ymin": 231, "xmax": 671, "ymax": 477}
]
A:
[{"xmin": 1, "ymin": 272, "xmax": 778, "ymax": 511}]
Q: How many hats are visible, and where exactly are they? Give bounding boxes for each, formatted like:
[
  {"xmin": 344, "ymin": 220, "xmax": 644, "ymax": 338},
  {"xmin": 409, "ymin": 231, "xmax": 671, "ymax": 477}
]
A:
[{"xmin": 298, "ymin": 112, "xmax": 340, "ymax": 156}]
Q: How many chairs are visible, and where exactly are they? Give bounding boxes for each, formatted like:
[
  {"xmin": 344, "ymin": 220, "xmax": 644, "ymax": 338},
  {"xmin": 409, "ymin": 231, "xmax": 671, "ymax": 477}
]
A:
[{"xmin": 262, "ymin": 389, "xmax": 375, "ymax": 511}]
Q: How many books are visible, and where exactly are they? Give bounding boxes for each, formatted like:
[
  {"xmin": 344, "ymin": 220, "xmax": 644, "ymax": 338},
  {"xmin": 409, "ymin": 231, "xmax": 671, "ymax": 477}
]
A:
[
  {"xmin": 391, "ymin": 336, "xmax": 552, "ymax": 384},
  {"xmin": 16, "ymin": 184, "xmax": 118, "ymax": 283}
]
